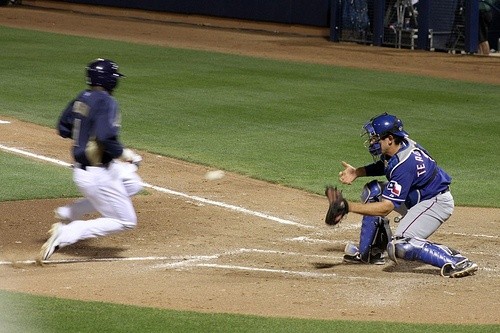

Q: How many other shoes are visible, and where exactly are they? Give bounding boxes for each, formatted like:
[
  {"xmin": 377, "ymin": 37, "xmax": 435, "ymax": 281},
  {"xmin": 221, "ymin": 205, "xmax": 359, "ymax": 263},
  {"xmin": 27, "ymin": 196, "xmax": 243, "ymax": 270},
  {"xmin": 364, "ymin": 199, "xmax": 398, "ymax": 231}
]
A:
[
  {"xmin": 53, "ymin": 207, "xmax": 71, "ymax": 225},
  {"xmin": 39, "ymin": 222, "xmax": 65, "ymax": 262}
]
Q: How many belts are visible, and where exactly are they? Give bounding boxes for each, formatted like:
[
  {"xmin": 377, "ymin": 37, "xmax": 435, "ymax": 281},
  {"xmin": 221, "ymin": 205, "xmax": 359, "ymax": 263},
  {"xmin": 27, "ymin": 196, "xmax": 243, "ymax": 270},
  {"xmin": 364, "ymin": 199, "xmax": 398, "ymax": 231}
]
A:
[
  {"xmin": 441, "ymin": 187, "xmax": 450, "ymax": 194},
  {"xmin": 73, "ymin": 162, "xmax": 87, "ymax": 171}
]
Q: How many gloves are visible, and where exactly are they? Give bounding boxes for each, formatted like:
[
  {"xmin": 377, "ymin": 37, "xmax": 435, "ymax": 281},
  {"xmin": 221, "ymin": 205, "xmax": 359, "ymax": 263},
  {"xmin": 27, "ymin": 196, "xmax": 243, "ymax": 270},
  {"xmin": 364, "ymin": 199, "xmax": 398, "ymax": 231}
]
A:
[{"xmin": 122, "ymin": 148, "xmax": 143, "ymax": 164}]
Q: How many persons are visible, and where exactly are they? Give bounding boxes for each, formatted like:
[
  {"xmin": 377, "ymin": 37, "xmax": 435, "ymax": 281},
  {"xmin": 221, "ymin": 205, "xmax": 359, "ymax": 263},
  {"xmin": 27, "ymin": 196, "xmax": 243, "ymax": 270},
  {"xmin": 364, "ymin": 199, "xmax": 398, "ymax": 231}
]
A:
[
  {"xmin": 38, "ymin": 57, "xmax": 143, "ymax": 260},
  {"xmin": 390, "ymin": 0, "xmax": 419, "ymax": 34},
  {"xmin": 479, "ymin": 0, "xmax": 496, "ymax": 55},
  {"xmin": 325, "ymin": 112, "xmax": 478, "ymax": 278}
]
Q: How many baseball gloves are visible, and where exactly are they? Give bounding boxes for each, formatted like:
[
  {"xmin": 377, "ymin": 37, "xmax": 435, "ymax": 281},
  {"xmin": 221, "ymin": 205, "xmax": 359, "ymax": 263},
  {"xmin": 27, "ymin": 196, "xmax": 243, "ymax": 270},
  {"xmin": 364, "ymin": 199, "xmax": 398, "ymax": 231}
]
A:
[{"xmin": 324, "ymin": 185, "xmax": 348, "ymax": 226}]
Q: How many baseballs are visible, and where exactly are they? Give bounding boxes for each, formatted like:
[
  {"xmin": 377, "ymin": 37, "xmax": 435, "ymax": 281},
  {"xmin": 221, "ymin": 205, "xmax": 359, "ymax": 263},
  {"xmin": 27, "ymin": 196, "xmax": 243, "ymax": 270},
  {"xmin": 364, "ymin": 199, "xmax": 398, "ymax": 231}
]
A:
[{"xmin": 205, "ymin": 170, "xmax": 226, "ymax": 181}]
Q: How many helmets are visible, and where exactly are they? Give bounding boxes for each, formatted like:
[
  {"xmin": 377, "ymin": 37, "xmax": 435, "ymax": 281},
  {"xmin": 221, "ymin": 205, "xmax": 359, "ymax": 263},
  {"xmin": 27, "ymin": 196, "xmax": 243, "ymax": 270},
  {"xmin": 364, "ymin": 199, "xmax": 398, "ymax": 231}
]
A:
[
  {"xmin": 363, "ymin": 113, "xmax": 410, "ymax": 138},
  {"xmin": 84, "ymin": 57, "xmax": 127, "ymax": 92}
]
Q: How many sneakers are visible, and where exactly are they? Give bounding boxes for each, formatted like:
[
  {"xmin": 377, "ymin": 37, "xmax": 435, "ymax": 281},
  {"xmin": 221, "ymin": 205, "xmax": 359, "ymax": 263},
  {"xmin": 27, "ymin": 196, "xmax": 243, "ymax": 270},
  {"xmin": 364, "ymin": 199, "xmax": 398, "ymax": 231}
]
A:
[
  {"xmin": 343, "ymin": 251, "xmax": 386, "ymax": 265},
  {"xmin": 448, "ymin": 260, "xmax": 479, "ymax": 279}
]
